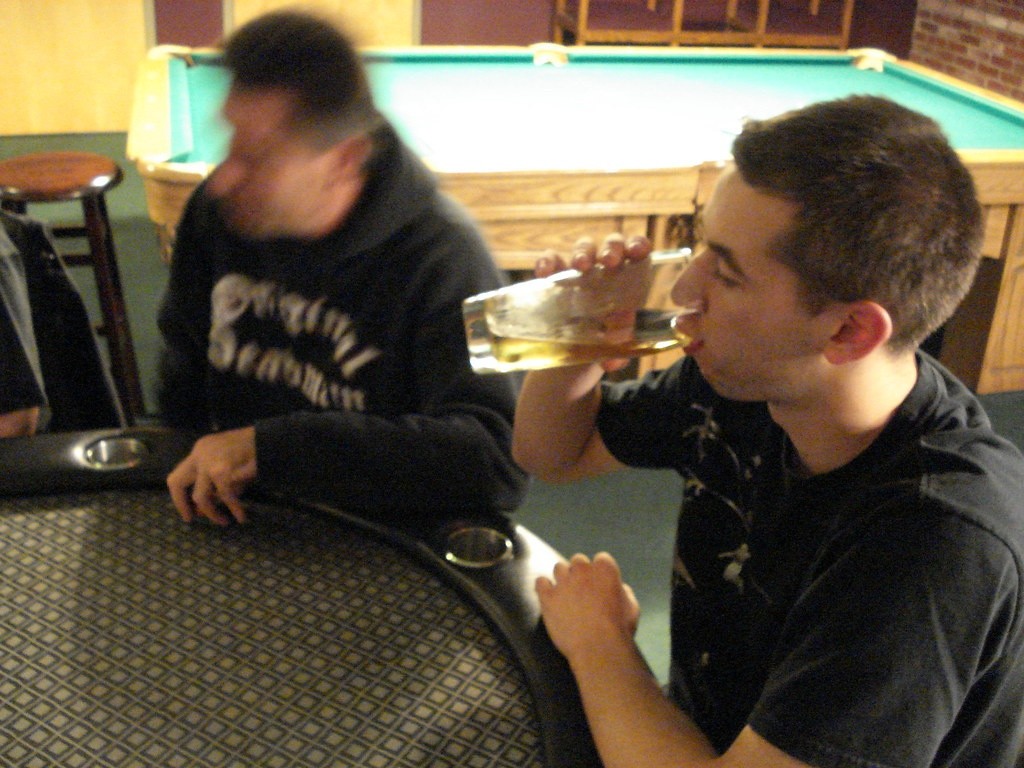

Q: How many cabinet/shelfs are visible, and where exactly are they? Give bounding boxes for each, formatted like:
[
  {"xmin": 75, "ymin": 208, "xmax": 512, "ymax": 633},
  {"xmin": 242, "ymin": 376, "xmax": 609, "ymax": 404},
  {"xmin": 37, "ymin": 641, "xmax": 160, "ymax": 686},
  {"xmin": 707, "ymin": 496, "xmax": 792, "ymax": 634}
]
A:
[{"xmin": 549, "ymin": 0, "xmax": 854, "ymax": 52}]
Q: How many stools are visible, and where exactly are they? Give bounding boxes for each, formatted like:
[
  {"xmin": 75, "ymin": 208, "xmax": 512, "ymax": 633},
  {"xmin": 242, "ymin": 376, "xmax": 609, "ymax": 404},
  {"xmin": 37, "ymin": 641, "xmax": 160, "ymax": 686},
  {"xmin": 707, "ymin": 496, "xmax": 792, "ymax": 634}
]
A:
[{"xmin": 0, "ymin": 152, "xmax": 144, "ymax": 421}]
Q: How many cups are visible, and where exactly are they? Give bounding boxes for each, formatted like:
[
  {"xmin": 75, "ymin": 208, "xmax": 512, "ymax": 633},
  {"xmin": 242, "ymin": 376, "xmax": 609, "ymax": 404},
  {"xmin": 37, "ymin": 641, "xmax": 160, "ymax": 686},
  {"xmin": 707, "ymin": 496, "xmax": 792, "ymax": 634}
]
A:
[{"xmin": 461, "ymin": 248, "xmax": 707, "ymax": 376}]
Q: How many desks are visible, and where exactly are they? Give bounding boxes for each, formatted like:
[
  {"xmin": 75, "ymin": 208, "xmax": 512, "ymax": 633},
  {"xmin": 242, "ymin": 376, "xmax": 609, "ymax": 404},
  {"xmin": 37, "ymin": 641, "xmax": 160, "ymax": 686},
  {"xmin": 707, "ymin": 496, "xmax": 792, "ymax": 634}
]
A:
[
  {"xmin": 0, "ymin": 428, "xmax": 600, "ymax": 768},
  {"xmin": 126, "ymin": 45, "xmax": 1024, "ymax": 397}
]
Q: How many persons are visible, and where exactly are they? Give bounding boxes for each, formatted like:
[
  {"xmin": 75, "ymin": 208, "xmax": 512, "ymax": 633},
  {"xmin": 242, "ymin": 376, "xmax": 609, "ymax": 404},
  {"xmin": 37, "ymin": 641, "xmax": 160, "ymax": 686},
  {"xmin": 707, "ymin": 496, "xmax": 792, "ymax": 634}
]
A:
[
  {"xmin": 513, "ymin": 93, "xmax": 1024, "ymax": 768},
  {"xmin": 150, "ymin": 7, "xmax": 531, "ymax": 527},
  {"xmin": 1, "ymin": 208, "xmax": 124, "ymax": 439}
]
